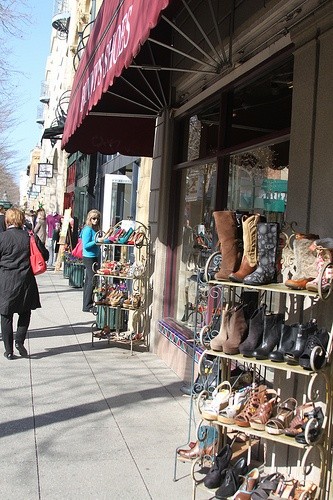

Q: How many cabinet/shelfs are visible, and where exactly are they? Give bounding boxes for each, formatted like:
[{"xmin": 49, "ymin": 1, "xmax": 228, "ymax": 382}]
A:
[
  {"xmin": 92, "ymin": 219, "xmax": 152, "ymax": 357},
  {"xmin": 193, "ymin": 251, "xmax": 333, "ymax": 500}
]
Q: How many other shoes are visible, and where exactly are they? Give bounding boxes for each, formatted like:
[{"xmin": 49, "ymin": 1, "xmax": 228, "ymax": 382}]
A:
[
  {"xmin": 83, "ymin": 306, "xmax": 97, "ymax": 312},
  {"xmin": 96, "ymin": 325, "xmax": 143, "ymax": 344},
  {"xmin": 4, "ymin": 352, "xmax": 13, "ymax": 360},
  {"xmin": 180, "ymin": 373, "xmax": 217, "ymax": 396},
  {"xmin": 15, "ymin": 342, "xmax": 27, "ymax": 358}
]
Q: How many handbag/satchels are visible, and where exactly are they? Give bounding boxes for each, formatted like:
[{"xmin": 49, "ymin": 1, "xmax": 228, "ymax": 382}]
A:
[
  {"xmin": 27, "ymin": 229, "xmax": 46, "ymax": 276},
  {"xmin": 71, "ymin": 239, "xmax": 82, "ymax": 259}
]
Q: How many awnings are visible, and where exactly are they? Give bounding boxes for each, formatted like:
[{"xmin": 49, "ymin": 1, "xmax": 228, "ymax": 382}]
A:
[{"xmin": 61, "ymin": 0, "xmax": 228, "ymax": 158}]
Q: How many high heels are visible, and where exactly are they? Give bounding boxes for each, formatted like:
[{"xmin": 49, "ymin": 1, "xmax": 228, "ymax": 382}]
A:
[
  {"xmin": 209, "ymin": 303, "xmax": 329, "ymax": 371},
  {"xmin": 95, "ymin": 287, "xmax": 142, "ymax": 308},
  {"xmin": 97, "ymin": 258, "xmax": 143, "ymax": 277},
  {"xmin": 97, "ymin": 226, "xmax": 146, "ymax": 245},
  {"xmin": 202, "ymin": 381, "xmax": 325, "ymax": 444},
  {"xmin": 178, "ymin": 432, "xmax": 318, "ymax": 500}
]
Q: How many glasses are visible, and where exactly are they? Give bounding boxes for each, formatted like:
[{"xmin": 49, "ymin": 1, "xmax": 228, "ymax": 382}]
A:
[{"xmin": 91, "ymin": 218, "xmax": 99, "ymax": 220}]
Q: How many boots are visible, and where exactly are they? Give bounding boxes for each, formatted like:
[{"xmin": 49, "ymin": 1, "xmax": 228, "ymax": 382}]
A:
[{"xmin": 212, "ymin": 210, "xmax": 333, "ymax": 292}]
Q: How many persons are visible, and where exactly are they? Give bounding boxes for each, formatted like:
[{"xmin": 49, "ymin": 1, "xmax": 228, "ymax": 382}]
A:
[
  {"xmin": 0, "ymin": 205, "xmax": 6, "ymax": 232},
  {"xmin": 81, "ymin": 209, "xmax": 101, "ymax": 313},
  {"xmin": 21, "ymin": 205, "xmax": 44, "ymax": 230},
  {"xmin": 0, "ymin": 208, "xmax": 50, "ymax": 360},
  {"xmin": 45, "ymin": 210, "xmax": 62, "ymax": 267},
  {"xmin": 33, "ymin": 210, "xmax": 46, "ymax": 245}
]
